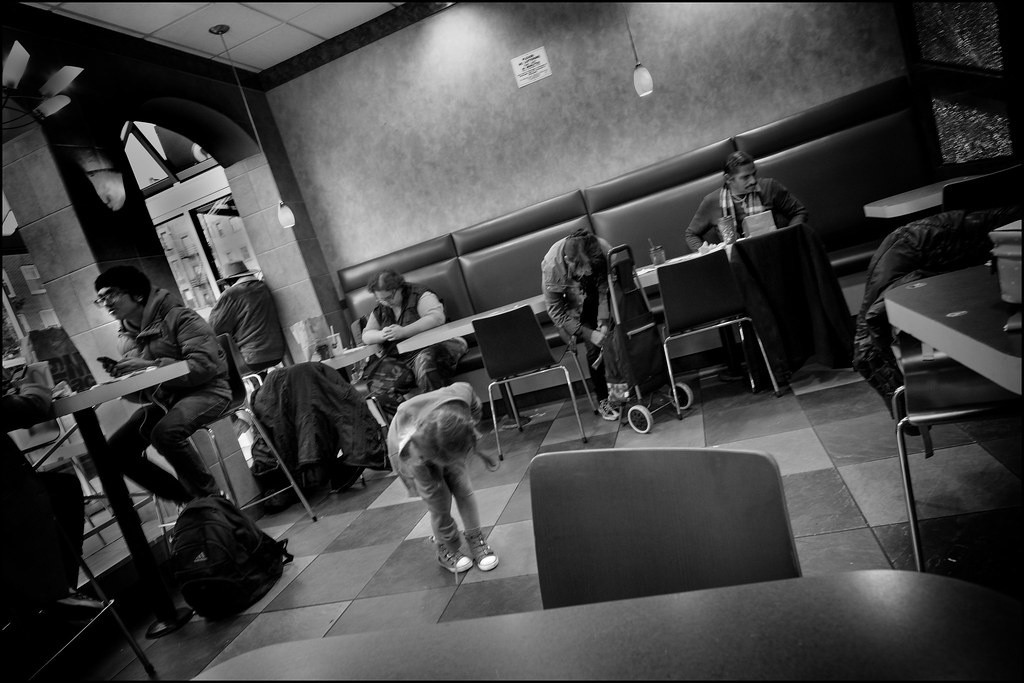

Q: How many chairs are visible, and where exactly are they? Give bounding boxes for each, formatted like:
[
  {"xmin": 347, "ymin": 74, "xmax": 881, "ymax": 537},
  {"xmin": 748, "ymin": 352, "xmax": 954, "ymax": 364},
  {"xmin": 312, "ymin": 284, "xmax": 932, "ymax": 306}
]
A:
[
  {"xmin": 873, "ymin": 211, "xmax": 1024, "ymax": 573},
  {"xmin": 471, "ymin": 304, "xmax": 587, "ymax": 461},
  {"xmin": 940, "ymin": 162, "xmax": 1024, "ymax": 213},
  {"xmin": 137, "ymin": 335, "xmax": 318, "ymax": 524},
  {"xmin": 654, "ymin": 222, "xmax": 853, "ymax": 421},
  {"xmin": 529, "ymin": 447, "xmax": 804, "ymax": 607},
  {"xmin": 225, "ymin": 333, "xmax": 280, "ymax": 390}
]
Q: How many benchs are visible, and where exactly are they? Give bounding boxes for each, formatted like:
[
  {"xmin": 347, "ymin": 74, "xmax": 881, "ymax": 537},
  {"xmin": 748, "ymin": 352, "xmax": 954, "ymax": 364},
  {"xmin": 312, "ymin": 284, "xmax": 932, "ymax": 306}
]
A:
[{"xmin": 330, "ymin": 71, "xmax": 943, "ymax": 404}]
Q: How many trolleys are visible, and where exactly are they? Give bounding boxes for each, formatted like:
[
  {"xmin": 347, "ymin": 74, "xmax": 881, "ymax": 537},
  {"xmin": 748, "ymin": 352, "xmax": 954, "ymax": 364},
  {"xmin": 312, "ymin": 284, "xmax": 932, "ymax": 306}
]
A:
[{"xmin": 599, "ymin": 243, "xmax": 694, "ymax": 434}]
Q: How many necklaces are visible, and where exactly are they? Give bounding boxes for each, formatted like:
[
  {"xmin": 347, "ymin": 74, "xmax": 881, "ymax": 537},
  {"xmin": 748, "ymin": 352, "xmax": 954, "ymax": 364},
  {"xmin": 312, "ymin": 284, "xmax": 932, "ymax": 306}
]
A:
[{"xmin": 731, "ymin": 192, "xmax": 748, "ymax": 214}]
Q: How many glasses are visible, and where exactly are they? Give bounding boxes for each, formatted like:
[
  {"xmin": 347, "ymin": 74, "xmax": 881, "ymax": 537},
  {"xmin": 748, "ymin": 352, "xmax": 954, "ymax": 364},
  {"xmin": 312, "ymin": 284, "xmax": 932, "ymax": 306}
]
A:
[
  {"xmin": 377, "ymin": 290, "xmax": 396, "ymax": 305},
  {"xmin": 94, "ymin": 290, "xmax": 116, "ymax": 309}
]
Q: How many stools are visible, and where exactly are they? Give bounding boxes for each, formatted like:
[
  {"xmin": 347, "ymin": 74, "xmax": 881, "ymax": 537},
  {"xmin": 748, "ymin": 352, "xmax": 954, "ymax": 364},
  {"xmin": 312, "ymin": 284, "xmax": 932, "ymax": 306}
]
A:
[{"xmin": 0, "ymin": 522, "xmax": 156, "ymax": 683}]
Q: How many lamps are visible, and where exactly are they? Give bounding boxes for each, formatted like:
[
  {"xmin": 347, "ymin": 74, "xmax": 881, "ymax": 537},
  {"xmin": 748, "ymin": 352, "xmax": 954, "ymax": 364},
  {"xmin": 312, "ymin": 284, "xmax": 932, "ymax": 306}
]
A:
[
  {"xmin": 0, "ymin": 38, "xmax": 84, "ymax": 128},
  {"xmin": 621, "ymin": 2, "xmax": 653, "ymax": 97},
  {"xmin": 209, "ymin": 24, "xmax": 295, "ymax": 228}
]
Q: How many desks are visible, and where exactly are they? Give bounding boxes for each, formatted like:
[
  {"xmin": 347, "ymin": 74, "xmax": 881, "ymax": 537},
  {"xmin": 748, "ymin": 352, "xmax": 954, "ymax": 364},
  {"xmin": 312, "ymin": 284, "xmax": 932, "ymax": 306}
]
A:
[
  {"xmin": 318, "ymin": 342, "xmax": 389, "ymax": 439},
  {"xmin": 396, "ymin": 237, "xmax": 737, "ymax": 428},
  {"xmin": 18, "ymin": 361, "xmax": 190, "ymax": 639},
  {"xmin": 883, "ymin": 266, "xmax": 1024, "ymax": 394},
  {"xmin": 864, "ymin": 175, "xmax": 988, "ymax": 218},
  {"xmin": 181, "ymin": 570, "xmax": 1024, "ymax": 683}
]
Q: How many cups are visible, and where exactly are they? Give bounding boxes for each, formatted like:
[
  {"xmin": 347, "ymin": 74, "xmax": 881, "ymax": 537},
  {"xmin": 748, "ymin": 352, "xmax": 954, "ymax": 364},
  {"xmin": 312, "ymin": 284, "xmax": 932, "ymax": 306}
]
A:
[
  {"xmin": 25, "ymin": 360, "xmax": 54, "ymax": 393},
  {"xmin": 649, "ymin": 246, "xmax": 666, "ymax": 265},
  {"xmin": 717, "ymin": 215, "xmax": 735, "ymax": 244},
  {"xmin": 326, "ymin": 332, "xmax": 344, "ymax": 356}
]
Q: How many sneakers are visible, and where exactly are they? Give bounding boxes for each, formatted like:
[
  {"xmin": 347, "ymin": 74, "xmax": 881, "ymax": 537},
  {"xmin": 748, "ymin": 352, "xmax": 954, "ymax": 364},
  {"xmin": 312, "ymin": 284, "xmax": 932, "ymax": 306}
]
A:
[
  {"xmin": 436, "ymin": 544, "xmax": 473, "ymax": 573},
  {"xmin": 468, "ymin": 536, "xmax": 499, "ymax": 572},
  {"xmin": 598, "ymin": 400, "xmax": 620, "ymax": 421},
  {"xmin": 53, "ymin": 589, "xmax": 104, "ymax": 608}
]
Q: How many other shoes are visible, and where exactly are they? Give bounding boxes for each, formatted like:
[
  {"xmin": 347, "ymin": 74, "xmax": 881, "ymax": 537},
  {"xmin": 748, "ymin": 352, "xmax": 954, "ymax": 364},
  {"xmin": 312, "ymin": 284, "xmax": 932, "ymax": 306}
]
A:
[
  {"xmin": 719, "ymin": 366, "xmax": 745, "ymax": 380},
  {"xmin": 176, "ymin": 475, "xmax": 219, "ymax": 508}
]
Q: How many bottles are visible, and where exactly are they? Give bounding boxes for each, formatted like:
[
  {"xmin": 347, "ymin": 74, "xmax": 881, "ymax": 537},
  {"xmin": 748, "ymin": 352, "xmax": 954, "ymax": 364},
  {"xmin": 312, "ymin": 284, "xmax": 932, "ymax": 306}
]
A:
[{"xmin": 315, "ymin": 338, "xmax": 330, "ymax": 359}]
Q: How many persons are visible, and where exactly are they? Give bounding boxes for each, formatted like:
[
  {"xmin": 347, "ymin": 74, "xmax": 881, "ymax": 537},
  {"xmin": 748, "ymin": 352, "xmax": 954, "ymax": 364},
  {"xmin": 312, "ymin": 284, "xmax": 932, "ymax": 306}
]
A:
[
  {"xmin": 541, "ymin": 228, "xmax": 621, "ymax": 422},
  {"xmin": 93, "ymin": 266, "xmax": 232, "ymax": 514},
  {"xmin": 0, "ymin": 383, "xmax": 104, "ymax": 609},
  {"xmin": 361, "ymin": 270, "xmax": 500, "ymax": 574},
  {"xmin": 208, "ymin": 259, "xmax": 285, "ymax": 388},
  {"xmin": 686, "ymin": 150, "xmax": 810, "ymax": 382}
]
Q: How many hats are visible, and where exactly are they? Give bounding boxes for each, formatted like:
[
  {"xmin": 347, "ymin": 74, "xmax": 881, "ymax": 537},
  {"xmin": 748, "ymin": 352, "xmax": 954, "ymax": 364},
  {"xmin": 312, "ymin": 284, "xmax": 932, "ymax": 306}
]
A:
[{"xmin": 216, "ymin": 261, "xmax": 255, "ymax": 285}]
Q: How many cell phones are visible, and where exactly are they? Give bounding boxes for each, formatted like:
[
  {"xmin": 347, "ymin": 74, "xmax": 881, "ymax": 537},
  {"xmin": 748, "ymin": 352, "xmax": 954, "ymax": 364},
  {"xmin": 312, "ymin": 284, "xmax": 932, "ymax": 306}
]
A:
[
  {"xmin": 9, "ymin": 365, "xmax": 28, "ymax": 382},
  {"xmin": 97, "ymin": 356, "xmax": 118, "ymax": 365}
]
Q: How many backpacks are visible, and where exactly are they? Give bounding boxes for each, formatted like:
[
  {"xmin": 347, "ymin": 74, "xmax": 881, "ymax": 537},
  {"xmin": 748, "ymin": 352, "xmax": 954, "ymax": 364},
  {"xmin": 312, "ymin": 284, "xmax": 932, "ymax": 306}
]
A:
[{"xmin": 167, "ymin": 493, "xmax": 294, "ymax": 625}]
